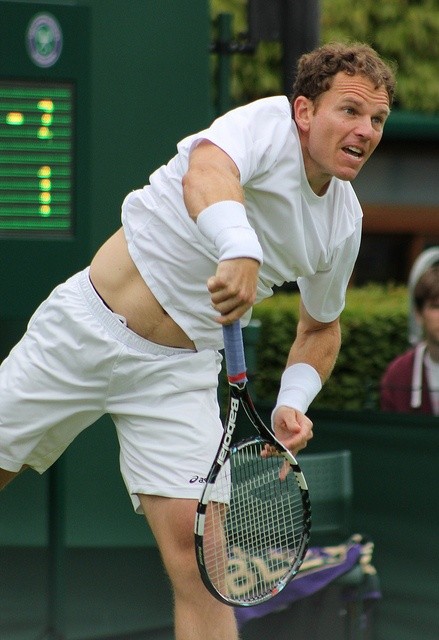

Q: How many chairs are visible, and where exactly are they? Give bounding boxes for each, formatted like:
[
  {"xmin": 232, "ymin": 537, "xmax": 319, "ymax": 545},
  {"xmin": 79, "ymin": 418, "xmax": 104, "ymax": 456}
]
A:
[{"xmin": 226, "ymin": 451, "xmax": 357, "ymax": 640}]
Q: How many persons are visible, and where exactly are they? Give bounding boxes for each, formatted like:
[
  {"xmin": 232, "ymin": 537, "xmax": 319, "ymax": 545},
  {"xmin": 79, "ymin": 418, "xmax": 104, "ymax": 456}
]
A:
[
  {"xmin": 0, "ymin": 38, "xmax": 397, "ymax": 640},
  {"xmin": 379, "ymin": 267, "xmax": 439, "ymax": 412}
]
